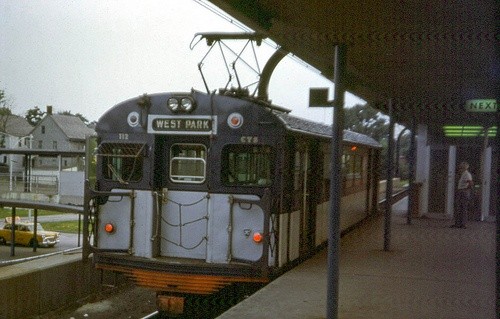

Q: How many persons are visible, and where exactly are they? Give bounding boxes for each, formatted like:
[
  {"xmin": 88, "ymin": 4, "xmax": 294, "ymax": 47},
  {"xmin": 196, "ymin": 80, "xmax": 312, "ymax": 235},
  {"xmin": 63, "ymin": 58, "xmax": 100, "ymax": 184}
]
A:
[{"xmin": 450, "ymin": 162, "xmax": 474, "ymax": 229}]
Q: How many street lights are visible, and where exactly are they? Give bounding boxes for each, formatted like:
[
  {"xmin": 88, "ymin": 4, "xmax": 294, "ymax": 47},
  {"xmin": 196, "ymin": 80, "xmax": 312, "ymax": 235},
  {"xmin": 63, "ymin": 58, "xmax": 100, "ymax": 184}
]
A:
[{"xmin": 10, "ymin": 135, "xmax": 34, "ymax": 192}]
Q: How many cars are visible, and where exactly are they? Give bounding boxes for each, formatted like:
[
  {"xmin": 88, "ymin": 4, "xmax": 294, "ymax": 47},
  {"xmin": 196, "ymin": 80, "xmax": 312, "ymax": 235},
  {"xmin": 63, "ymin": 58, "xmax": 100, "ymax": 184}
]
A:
[{"xmin": 0, "ymin": 216, "xmax": 61, "ymax": 248}]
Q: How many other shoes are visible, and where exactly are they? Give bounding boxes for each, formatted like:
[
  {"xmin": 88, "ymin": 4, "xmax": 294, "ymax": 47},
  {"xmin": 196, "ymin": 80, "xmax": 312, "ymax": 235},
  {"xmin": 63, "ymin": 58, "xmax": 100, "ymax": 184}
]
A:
[{"xmin": 451, "ymin": 224, "xmax": 464, "ymax": 228}]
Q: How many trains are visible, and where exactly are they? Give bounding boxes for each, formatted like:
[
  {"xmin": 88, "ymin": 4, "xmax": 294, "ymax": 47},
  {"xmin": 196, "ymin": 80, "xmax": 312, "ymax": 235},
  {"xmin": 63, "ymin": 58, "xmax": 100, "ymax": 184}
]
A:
[{"xmin": 93, "ymin": 89, "xmax": 385, "ymax": 296}]
